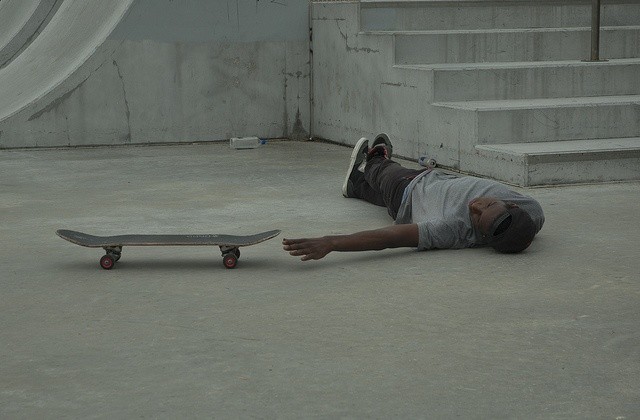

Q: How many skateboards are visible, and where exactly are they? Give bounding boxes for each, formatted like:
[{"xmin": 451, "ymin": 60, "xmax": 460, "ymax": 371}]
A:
[{"xmin": 54, "ymin": 229, "xmax": 281, "ymax": 271}]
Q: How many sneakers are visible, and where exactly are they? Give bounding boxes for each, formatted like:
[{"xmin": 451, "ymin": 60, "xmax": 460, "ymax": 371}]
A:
[
  {"xmin": 342, "ymin": 137, "xmax": 369, "ymax": 198},
  {"xmin": 371, "ymin": 132, "xmax": 394, "ymax": 159}
]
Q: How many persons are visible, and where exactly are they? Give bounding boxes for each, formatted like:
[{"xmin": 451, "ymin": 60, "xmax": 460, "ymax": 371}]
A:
[{"xmin": 283, "ymin": 133, "xmax": 545, "ymax": 261}]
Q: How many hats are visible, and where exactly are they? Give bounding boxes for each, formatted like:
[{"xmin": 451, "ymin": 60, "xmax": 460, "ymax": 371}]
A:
[{"xmin": 484, "ymin": 209, "xmax": 537, "ymax": 254}]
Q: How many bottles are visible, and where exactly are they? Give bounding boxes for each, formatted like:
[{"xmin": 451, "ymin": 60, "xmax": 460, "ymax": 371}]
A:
[{"xmin": 230, "ymin": 135, "xmax": 265, "ymax": 150}]
